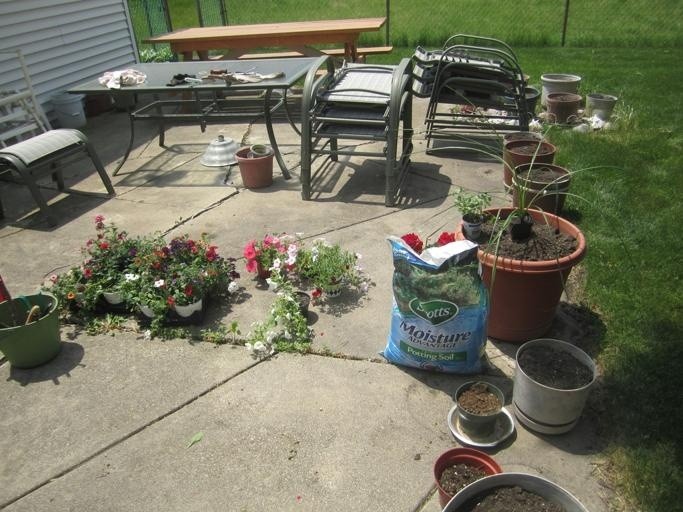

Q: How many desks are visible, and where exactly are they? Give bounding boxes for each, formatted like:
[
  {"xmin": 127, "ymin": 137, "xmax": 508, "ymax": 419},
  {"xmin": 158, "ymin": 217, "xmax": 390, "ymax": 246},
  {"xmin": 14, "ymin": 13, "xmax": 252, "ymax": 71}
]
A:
[
  {"xmin": 141, "ymin": 17, "xmax": 386, "ymax": 113},
  {"xmin": 64, "ymin": 56, "xmax": 321, "ymax": 179}
]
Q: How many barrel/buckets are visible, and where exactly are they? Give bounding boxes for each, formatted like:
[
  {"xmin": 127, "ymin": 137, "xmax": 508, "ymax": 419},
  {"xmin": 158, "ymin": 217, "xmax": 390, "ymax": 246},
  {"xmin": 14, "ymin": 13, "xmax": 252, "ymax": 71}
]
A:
[{"xmin": 585, "ymin": 92, "xmax": 618, "ymax": 123}]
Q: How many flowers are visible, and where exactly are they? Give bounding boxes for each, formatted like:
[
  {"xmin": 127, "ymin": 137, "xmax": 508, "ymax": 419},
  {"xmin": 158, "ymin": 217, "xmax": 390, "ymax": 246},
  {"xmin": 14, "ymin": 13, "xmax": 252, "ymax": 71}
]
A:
[
  {"xmin": 245, "ymin": 232, "xmax": 375, "ymax": 360},
  {"xmin": 41, "ymin": 214, "xmax": 239, "ymax": 319}
]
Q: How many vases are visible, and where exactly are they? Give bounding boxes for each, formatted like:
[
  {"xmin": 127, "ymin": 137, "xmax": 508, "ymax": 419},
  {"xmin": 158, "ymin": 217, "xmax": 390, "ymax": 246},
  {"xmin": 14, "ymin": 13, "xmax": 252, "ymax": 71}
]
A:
[
  {"xmin": 250, "ymin": 144, "xmax": 270, "ymax": 158},
  {"xmin": 520, "ymin": 72, "xmax": 618, "ymax": 126},
  {"xmin": 459, "ymin": 206, "xmax": 586, "ymax": 343},
  {"xmin": 234, "ymin": 144, "xmax": 274, "ymax": 188},
  {"xmin": 454, "ymin": 381, "xmax": 505, "ymax": 435},
  {"xmin": 502, "ymin": 140, "xmax": 556, "ymax": 194},
  {"xmin": 511, "ymin": 163, "xmax": 571, "ymax": 217},
  {"xmin": 434, "ymin": 447, "xmax": 503, "ymax": 510},
  {"xmin": 444, "ymin": 472, "xmax": 590, "ymax": 512},
  {"xmin": 514, "ymin": 338, "xmax": 598, "ymax": 435},
  {"xmin": 502, "ymin": 133, "xmax": 548, "ymax": 146}
]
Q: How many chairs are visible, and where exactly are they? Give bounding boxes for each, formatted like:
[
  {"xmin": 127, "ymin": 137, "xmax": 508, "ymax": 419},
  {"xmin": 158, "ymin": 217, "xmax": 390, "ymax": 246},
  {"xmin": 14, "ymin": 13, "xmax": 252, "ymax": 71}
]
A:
[
  {"xmin": 411, "ymin": 34, "xmax": 533, "ymax": 153},
  {"xmin": 1, "ymin": 49, "xmax": 114, "ymax": 227},
  {"xmin": 301, "ymin": 54, "xmax": 413, "ymax": 206}
]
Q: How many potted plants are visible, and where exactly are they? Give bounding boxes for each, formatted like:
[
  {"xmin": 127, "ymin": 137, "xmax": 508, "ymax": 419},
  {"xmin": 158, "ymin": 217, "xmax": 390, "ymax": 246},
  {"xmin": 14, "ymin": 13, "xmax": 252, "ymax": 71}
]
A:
[
  {"xmin": 455, "ymin": 190, "xmax": 493, "ymax": 243},
  {"xmin": 414, "ymin": 79, "xmax": 648, "ymax": 314}
]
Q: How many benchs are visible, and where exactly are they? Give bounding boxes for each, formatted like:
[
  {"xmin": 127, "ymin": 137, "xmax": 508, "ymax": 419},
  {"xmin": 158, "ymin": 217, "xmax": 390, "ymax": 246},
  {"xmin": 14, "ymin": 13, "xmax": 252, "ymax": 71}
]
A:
[{"xmin": 203, "ymin": 47, "xmax": 393, "ymax": 80}]
